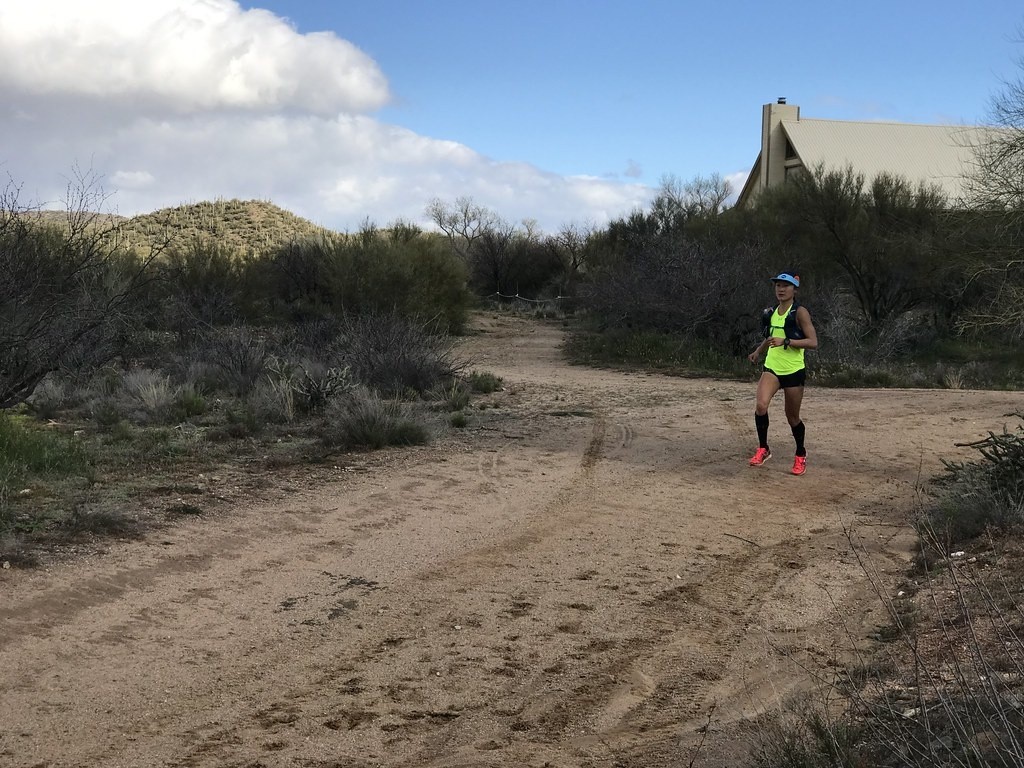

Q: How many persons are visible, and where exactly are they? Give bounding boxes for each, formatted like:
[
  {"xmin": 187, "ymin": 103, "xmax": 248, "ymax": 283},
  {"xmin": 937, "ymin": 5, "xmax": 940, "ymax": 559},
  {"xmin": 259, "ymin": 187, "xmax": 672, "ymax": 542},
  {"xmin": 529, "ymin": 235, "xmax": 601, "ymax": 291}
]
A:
[{"xmin": 748, "ymin": 272, "xmax": 818, "ymax": 476}]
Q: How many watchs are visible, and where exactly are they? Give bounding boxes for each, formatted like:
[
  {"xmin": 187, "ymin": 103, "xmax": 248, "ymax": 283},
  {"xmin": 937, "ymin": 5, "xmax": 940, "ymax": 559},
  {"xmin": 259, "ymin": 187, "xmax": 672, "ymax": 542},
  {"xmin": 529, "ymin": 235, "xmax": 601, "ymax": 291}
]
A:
[{"xmin": 784, "ymin": 338, "xmax": 790, "ymax": 350}]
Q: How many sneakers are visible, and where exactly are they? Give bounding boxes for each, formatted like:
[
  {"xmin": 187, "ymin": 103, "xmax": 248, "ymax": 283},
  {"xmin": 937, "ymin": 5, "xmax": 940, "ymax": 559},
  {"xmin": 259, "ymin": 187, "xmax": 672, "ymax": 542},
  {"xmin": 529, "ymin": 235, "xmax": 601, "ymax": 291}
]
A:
[
  {"xmin": 750, "ymin": 448, "xmax": 772, "ymax": 467},
  {"xmin": 792, "ymin": 455, "xmax": 807, "ymax": 475}
]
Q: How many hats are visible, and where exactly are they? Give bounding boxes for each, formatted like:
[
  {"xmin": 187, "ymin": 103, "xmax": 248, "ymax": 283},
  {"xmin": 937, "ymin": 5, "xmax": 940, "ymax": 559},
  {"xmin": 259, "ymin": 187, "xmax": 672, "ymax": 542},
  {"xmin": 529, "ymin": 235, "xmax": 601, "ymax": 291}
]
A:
[{"xmin": 770, "ymin": 271, "xmax": 800, "ymax": 288}]
